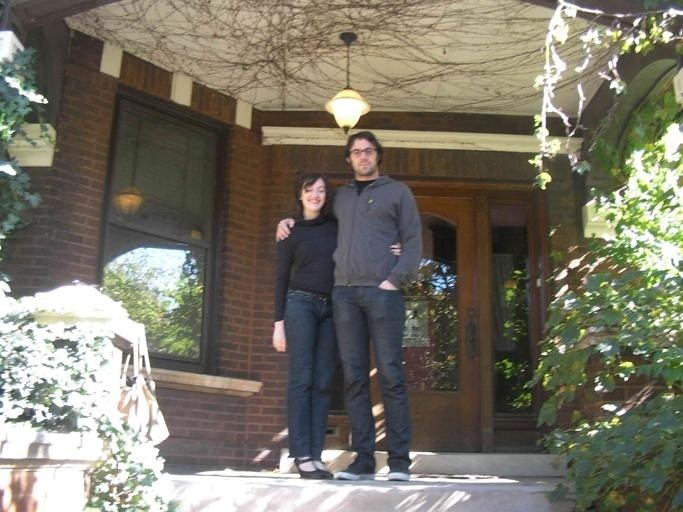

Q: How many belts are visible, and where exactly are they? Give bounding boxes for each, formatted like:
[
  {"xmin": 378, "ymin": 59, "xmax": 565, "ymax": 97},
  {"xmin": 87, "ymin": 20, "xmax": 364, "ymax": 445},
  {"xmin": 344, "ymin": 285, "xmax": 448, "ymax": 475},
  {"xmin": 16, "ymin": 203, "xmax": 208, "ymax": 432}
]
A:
[{"xmin": 288, "ymin": 287, "xmax": 332, "ymax": 304}]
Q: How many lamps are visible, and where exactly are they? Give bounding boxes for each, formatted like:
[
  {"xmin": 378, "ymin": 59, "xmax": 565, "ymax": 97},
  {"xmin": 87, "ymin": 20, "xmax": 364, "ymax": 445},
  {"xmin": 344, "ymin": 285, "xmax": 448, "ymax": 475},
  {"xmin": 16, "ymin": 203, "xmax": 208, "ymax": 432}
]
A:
[
  {"xmin": 325, "ymin": 32, "xmax": 372, "ymax": 135},
  {"xmin": 113, "ymin": 118, "xmax": 144, "ymax": 220}
]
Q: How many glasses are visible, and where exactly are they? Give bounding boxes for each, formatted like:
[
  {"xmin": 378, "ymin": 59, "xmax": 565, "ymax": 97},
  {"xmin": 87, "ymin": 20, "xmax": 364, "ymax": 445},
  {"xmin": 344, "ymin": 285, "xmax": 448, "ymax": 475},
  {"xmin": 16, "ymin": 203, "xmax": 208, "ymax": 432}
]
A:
[{"xmin": 347, "ymin": 146, "xmax": 381, "ymax": 157}]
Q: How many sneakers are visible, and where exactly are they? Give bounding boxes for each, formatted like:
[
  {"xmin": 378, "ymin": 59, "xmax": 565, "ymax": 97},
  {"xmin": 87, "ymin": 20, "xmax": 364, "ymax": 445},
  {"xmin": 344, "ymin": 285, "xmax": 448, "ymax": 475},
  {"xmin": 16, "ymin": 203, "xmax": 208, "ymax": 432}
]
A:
[
  {"xmin": 387, "ymin": 461, "xmax": 413, "ymax": 482},
  {"xmin": 335, "ymin": 457, "xmax": 377, "ymax": 481}
]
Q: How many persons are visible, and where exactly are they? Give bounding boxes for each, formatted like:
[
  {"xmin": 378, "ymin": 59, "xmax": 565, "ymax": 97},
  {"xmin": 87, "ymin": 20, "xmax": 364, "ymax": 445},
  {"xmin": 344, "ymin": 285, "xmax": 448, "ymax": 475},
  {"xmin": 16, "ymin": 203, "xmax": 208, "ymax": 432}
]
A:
[
  {"xmin": 275, "ymin": 132, "xmax": 424, "ymax": 480},
  {"xmin": 269, "ymin": 173, "xmax": 402, "ymax": 479}
]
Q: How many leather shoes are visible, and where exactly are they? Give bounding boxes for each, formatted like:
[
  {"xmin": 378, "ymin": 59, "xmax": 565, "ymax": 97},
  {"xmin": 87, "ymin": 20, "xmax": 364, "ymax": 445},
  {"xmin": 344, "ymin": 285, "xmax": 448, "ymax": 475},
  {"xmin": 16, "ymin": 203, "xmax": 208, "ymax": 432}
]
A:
[{"xmin": 294, "ymin": 454, "xmax": 334, "ymax": 479}]
